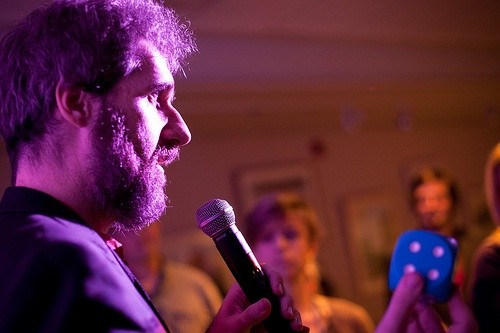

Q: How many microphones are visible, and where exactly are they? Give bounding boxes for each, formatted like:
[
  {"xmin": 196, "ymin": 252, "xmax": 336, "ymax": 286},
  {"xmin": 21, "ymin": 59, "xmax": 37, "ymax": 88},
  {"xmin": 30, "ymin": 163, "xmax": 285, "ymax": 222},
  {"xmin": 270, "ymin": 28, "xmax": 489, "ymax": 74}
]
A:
[{"xmin": 196, "ymin": 199, "xmax": 297, "ymax": 333}]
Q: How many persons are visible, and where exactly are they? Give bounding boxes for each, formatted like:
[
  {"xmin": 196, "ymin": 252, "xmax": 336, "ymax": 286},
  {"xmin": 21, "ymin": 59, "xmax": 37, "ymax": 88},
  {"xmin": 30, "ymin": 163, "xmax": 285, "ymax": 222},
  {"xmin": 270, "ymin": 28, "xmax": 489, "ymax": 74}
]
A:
[
  {"xmin": 108, "ymin": 142, "xmax": 500, "ymax": 333},
  {"xmin": 0, "ymin": 0, "xmax": 311, "ymax": 333}
]
